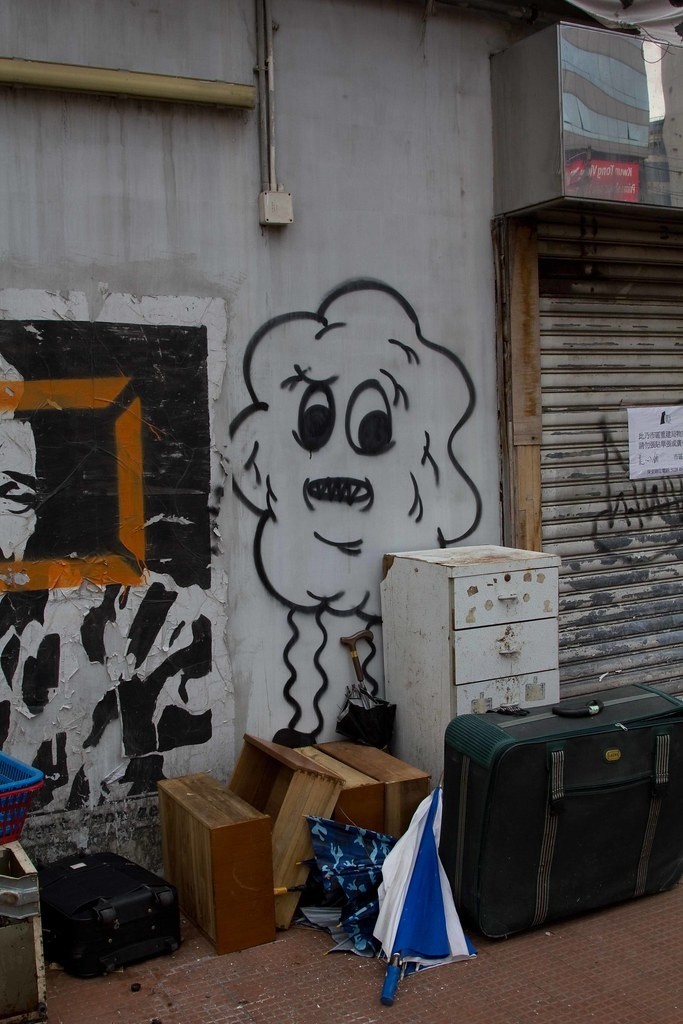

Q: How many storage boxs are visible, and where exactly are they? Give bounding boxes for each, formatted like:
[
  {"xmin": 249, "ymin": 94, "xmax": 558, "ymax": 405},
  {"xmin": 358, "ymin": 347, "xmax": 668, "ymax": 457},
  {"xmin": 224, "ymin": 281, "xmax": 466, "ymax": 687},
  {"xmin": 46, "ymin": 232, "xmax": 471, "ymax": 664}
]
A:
[{"xmin": 157, "ymin": 733, "xmax": 434, "ymax": 958}]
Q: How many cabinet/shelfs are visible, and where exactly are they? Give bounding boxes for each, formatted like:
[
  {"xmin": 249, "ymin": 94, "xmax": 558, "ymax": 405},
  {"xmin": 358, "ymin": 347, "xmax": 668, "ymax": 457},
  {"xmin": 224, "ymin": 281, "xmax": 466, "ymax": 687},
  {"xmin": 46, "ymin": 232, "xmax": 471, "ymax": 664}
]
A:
[{"xmin": 379, "ymin": 545, "xmax": 562, "ymax": 787}]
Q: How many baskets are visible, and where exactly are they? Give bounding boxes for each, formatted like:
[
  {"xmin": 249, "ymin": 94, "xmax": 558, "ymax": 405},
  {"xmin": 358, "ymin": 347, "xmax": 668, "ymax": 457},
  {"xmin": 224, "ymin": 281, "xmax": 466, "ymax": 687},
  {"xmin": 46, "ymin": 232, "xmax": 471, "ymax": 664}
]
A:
[{"xmin": 0, "ymin": 750, "xmax": 45, "ymax": 845}]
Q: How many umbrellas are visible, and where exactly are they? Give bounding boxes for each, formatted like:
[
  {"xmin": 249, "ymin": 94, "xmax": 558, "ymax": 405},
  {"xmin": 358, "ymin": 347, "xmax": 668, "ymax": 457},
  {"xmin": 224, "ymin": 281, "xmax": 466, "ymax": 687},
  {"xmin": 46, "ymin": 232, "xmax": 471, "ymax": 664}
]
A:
[
  {"xmin": 335, "ymin": 629, "xmax": 397, "ymax": 756},
  {"xmin": 272, "ymin": 769, "xmax": 479, "ymax": 1007}
]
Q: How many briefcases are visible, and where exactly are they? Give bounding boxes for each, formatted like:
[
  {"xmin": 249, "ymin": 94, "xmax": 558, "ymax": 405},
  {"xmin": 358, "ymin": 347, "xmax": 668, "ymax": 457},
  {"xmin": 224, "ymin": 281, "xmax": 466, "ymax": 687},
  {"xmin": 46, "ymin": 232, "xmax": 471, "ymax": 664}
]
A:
[{"xmin": 437, "ymin": 681, "xmax": 683, "ymax": 940}]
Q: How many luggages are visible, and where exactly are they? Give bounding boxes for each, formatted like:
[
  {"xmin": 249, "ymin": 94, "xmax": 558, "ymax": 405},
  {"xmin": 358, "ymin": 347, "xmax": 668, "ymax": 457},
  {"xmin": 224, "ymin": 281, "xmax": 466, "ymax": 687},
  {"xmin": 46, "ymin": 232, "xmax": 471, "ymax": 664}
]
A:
[{"xmin": 38, "ymin": 852, "xmax": 181, "ymax": 979}]
